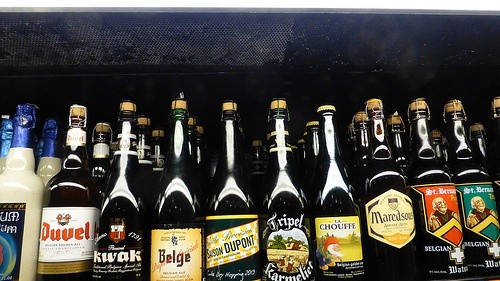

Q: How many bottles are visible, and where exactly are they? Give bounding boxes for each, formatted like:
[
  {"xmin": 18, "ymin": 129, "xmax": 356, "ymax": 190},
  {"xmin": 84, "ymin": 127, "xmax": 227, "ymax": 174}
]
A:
[{"xmin": 0, "ymin": 98, "xmax": 500, "ymax": 281}]
